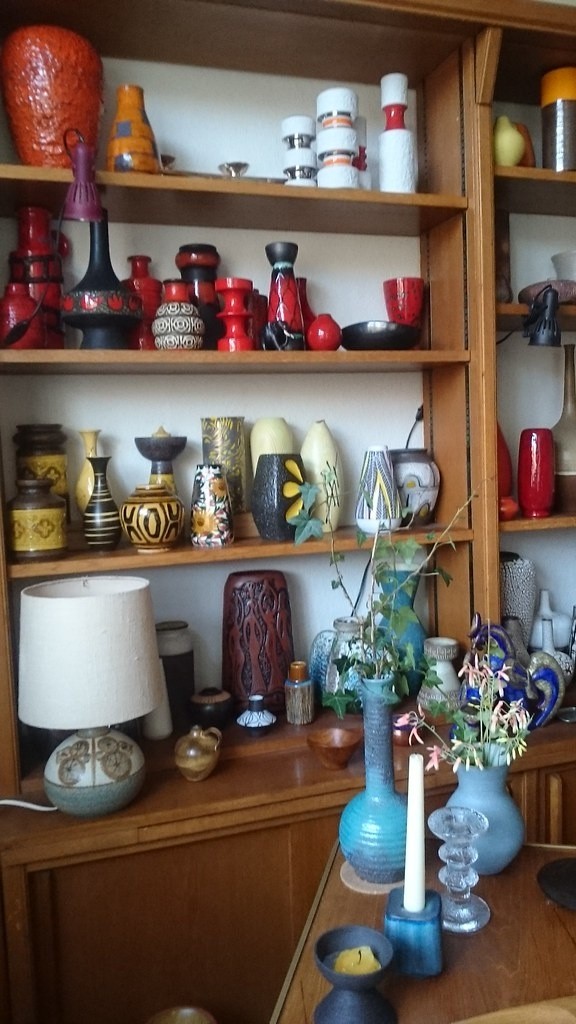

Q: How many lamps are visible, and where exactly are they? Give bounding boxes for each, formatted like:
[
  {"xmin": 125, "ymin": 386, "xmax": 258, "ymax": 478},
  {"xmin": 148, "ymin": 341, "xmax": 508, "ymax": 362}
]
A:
[{"xmin": 16, "ymin": 574, "xmax": 167, "ymax": 818}]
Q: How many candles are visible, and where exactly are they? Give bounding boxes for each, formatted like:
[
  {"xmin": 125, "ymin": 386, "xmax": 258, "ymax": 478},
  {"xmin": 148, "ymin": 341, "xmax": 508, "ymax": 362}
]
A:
[{"xmin": 404, "ymin": 751, "xmax": 426, "ymax": 912}]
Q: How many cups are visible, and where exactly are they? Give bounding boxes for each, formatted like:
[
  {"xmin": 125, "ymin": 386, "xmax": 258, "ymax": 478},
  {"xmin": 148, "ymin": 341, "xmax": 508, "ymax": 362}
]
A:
[
  {"xmin": 384, "ymin": 277, "xmax": 425, "ymax": 330},
  {"xmin": 391, "ymin": 714, "xmax": 425, "ymax": 746},
  {"xmin": 201, "ymin": 418, "xmax": 249, "ymax": 513},
  {"xmin": 306, "ymin": 726, "xmax": 365, "ymax": 771}
]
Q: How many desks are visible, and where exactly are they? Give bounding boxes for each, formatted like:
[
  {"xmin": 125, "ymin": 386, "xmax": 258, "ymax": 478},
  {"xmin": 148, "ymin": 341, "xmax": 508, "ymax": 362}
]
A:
[{"xmin": 272, "ymin": 839, "xmax": 576, "ymax": 1024}]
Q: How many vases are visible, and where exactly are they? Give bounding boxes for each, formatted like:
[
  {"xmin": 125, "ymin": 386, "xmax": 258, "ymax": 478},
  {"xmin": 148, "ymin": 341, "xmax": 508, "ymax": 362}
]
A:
[
  {"xmin": 444, "ymin": 741, "xmax": 523, "ymax": 876},
  {"xmin": 11, "ymin": 345, "xmax": 576, "ymax": 883}
]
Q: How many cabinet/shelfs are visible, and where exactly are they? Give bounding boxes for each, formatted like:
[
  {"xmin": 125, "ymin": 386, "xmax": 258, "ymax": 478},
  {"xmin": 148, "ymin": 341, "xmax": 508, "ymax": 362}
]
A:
[
  {"xmin": 429, "ymin": 0, "xmax": 576, "ymax": 864},
  {"xmin": 0, "ymin": 0, "xmax": 469, "ymax": 1024}
]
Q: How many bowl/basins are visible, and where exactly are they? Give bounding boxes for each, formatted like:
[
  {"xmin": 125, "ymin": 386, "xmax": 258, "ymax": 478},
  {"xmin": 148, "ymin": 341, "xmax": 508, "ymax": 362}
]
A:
[
  {"xmin": 551, "ymin": 250, "xmax": 576, "ymax": 285},
  {"xmin": 343, "ymin": 321, "xmax": 420, "ymax": 350}
]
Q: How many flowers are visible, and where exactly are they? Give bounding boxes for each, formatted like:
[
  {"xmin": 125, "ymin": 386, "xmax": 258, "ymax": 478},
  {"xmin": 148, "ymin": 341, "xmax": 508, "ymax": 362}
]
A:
[{"xmin": 394, "ymin": 652, "xmax": 532, "ymax": 772}]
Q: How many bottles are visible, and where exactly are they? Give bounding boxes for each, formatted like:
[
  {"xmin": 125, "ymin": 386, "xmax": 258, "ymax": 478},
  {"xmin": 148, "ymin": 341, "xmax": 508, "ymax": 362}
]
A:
[
  {"xmin": 497, "ymin": 344, "xmax": 576, "ymax": 520},
  {"xmin": 174, "ymin": 724, "xmax": 222, "ymax": 781},
  {"xmin": 492, "ymin": 66, "xmax": 576, "ymax": 172},
  {"xmin": 4, "ymin": 415, "xmax": 441, "ymax": 561},
  {"xmin": 107, "ymin": 84, "xmax": 159, "ymax": 173},
  {"xmin": 0, "ymin": 22, "xmax": 103, "ymax": 168},
  {"xmin": 0, "ymin": 208, "xmax": 343, "ymax": 351},
  {"xmin": 139, "ymin": 568, "xmax": 460, "ymax": 742},
  {"xmin": 498, "ymin": 552, "xmax": 576, "ymax": 688}
]
